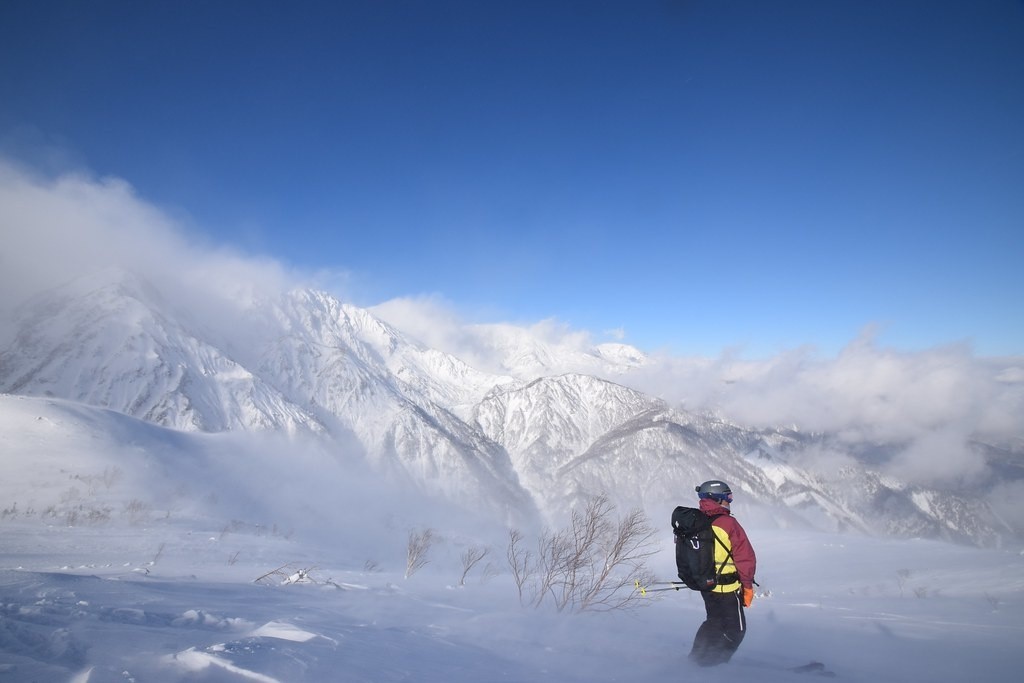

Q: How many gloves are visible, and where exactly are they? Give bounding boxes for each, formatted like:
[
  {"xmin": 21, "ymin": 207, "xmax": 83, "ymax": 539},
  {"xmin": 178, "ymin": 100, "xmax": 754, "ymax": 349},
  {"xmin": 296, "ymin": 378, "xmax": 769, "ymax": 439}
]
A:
[{"xmin": 742, "ymin": 588, "xmax": 755, "ymax": 608}]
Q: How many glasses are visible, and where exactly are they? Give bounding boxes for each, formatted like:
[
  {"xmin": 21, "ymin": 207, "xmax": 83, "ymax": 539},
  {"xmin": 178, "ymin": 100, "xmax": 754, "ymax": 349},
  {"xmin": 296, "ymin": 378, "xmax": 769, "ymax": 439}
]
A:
[{"xmin": 724, "ymin": 492, "xmax": 733, "ymax": 502}]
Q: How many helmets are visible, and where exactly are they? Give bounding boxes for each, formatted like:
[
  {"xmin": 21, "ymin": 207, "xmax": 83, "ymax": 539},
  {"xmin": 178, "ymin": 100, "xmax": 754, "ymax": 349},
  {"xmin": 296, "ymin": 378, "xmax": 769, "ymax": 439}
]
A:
[{"xmin": 696, "ymin": 480, "xmax": 731, "ymax": 497}]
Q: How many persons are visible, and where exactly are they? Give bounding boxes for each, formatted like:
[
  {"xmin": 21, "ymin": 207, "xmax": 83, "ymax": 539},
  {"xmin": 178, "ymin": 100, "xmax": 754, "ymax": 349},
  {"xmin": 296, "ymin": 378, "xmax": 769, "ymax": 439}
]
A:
[{"xmin": 687, "ymin": 480, "xmax": 757, "ymax": 667}]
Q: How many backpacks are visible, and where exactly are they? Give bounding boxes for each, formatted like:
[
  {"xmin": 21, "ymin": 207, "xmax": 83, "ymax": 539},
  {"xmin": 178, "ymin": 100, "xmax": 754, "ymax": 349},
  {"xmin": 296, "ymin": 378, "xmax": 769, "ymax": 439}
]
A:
[{"xmin": 671, "ymin": 506, "xmax": 739, "ymax": 591}]
